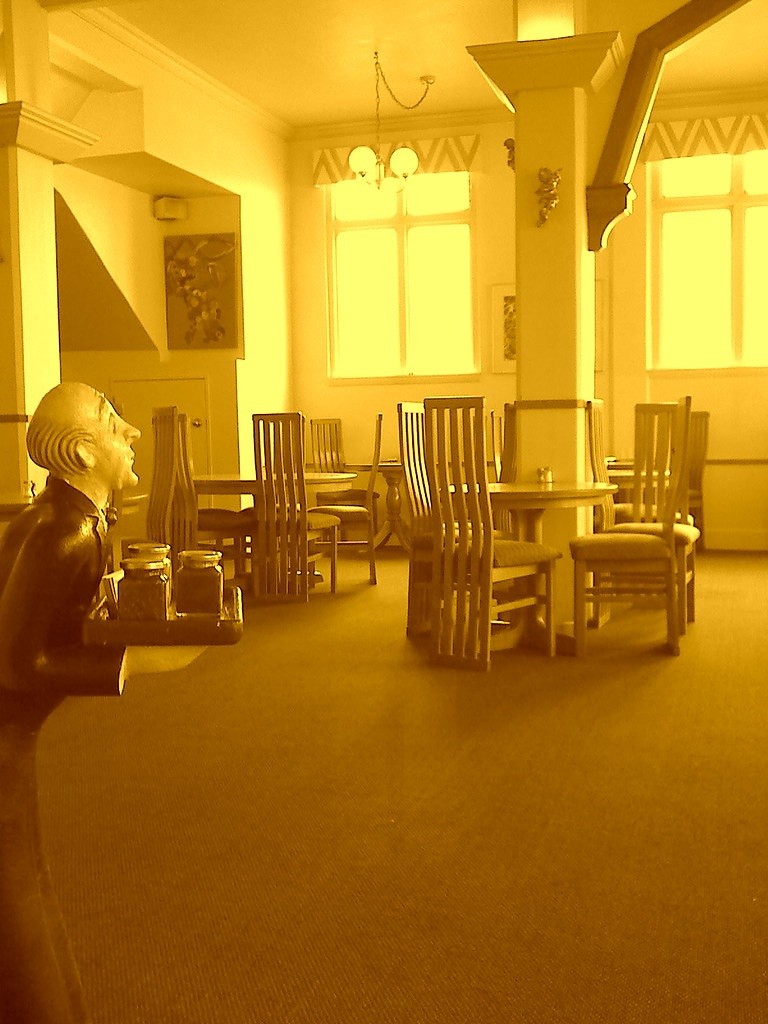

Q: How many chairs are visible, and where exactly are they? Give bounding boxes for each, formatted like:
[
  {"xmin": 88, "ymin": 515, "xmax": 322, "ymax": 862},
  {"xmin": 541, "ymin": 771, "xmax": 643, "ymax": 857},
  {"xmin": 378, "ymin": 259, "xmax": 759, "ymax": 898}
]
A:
[
  {"xmin": 397, "ymin": 397, "xmax": 562, "ymax": 669},
  {"xmin": 569, "ymin": 395, "xmax": 711, "ymax": 656},
  {"xmin": 147, "ymin": 406, "xmax": 380, "ymax": 602}
]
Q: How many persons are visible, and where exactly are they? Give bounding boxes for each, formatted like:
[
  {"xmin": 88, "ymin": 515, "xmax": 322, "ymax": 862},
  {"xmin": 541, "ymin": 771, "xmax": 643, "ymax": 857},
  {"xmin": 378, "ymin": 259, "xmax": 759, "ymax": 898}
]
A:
[{"xmin": 0, "ymin": 381, "xmax": 209, "ymax": 1024}]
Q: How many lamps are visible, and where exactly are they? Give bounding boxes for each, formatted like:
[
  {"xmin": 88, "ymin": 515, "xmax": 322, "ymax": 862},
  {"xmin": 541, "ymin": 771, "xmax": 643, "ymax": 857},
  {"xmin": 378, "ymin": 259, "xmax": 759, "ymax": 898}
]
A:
[{"xmin": 348, "ymin": 52, "xmax": 436, "ymax": 196}]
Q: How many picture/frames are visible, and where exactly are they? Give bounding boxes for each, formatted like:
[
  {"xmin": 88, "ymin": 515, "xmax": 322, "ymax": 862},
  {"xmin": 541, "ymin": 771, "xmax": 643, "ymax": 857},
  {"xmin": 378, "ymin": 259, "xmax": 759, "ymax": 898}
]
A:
[
  {"xmin": 492, "ymin": 284, "xmax": 515, "ymax": 371},
  {"xmin": 164, "ymin": 232, "xmax": 237, "ymax": 350}
]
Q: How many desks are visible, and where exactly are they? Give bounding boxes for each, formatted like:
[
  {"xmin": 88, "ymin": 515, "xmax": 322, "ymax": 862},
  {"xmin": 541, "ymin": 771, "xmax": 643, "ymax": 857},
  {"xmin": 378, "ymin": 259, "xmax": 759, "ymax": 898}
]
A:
[
  {"xmin": 193, "ymin": 472, "xmax": 358, "ymax": 599},
  {"xmin": 606, "ymin": 459, "xmax": 670, "ymax": 502},
  {"xmin": 439, "ymin": 482, "xmax": 619, "ymax": 656},
  {"xmin": 306, "ymin": 459, "xmax": 412, "ymax": 557}
]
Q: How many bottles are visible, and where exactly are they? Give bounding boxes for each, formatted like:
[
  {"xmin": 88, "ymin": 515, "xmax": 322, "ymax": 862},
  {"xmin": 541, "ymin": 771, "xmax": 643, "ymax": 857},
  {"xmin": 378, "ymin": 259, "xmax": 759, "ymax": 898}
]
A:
[
  {"xmin": 537, "ymin": 466, "xmax": 552, "ymax": 482},
  {"xmin": 116, "ymin": 541, "xmax": 225, "ymax": 620}
]
